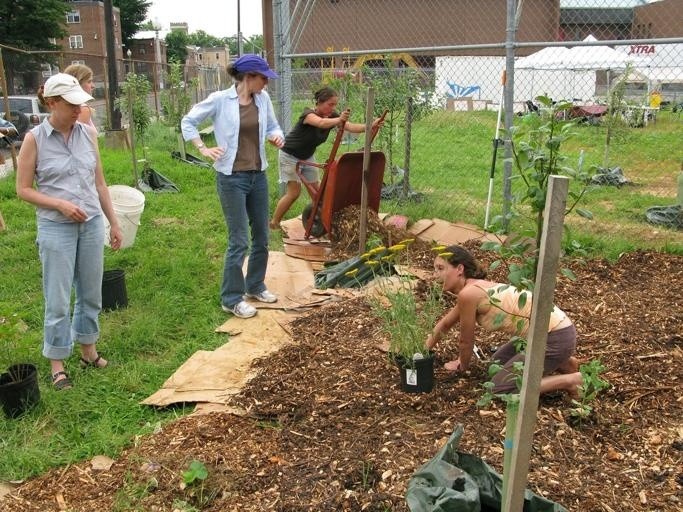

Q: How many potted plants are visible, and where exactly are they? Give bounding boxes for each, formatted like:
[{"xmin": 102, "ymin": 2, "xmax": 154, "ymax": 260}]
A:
[{"xmin": 0, "ymin": 329, "xmax": 40, "ymax": 419}]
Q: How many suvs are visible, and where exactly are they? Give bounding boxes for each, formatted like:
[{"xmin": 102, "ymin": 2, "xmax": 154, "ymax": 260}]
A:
[{"xmin": 0, "ymin": 93, "xmax": 48, "ymax": 145}]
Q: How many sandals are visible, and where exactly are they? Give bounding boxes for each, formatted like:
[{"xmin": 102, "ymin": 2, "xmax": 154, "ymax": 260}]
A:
[
  {"xmin": 51, "ymin": 370, "xmax": 73, "ymax": 392},
  {"xmin": 79, "ymin": 356, "xmax": 119, "ymax": 370}
]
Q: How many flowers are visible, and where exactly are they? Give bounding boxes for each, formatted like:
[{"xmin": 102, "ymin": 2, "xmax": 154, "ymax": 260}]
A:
[{"xmin": 344, "ymin": 239, "xmax": 453, "ymax": 380}]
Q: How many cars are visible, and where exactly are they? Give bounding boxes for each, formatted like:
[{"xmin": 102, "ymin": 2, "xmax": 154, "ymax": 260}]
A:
[{"xmin": 170, "ymin": 80, "xmax": 189, "ymax": 94}]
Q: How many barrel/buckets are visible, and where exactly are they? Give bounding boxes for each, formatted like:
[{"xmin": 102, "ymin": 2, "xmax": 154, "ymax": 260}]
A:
[{"xmin": 102, "ymin": 185, "xmax": 145, "ymax": 249}]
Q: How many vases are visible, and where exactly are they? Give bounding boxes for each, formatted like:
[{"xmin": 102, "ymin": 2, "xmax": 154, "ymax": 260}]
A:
[
  {"xmin": 388, "ymin": 351, "xmax": 435, "ymax": 393},
  {"xmin": 102, "ymin": 270, "xmax": 128, "ymax": 312}
]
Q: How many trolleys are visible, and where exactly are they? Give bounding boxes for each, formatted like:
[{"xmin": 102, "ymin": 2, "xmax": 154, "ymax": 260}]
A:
[{"xmin": 296, "ymin": 108, "xmax": 389, "ymax": 240}]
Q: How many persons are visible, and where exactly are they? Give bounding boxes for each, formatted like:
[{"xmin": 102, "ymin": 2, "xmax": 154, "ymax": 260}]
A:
[
  {"xmin": 16, "ymin": 73, "xmax": 123, "ymax": 394},
  {"xmin": 63, "ymin": 63, "xmax": 97, "ymax": 131},
  {"xmin": 268, "ymin": 85, "xmax": 385, "ymax": 239},
  {"xmin": 374, "ymin": 243, "xmax": 586, "ymax": 406},
  {"xmin": 180, "ymin": 53, "xmax": 285, "ymax": 319}
]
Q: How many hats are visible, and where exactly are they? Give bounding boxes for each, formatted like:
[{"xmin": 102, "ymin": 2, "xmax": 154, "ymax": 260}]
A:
[
  {"xmin": 42, "ymin": 72, "xmax": 95, "ymax": 105},
  {"xmin": 233, "ymin": 54, "xmax": 279, "ymax": 80}
]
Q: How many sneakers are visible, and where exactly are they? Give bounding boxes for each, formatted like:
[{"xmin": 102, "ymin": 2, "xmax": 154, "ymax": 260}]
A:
[
  {"xmin": 244, "ymin": 289, "xmax": 277, "ymax": 304},
  {"xmin": 221, "ymin": 301, "xmax": 257, "ymax": 319}
]
